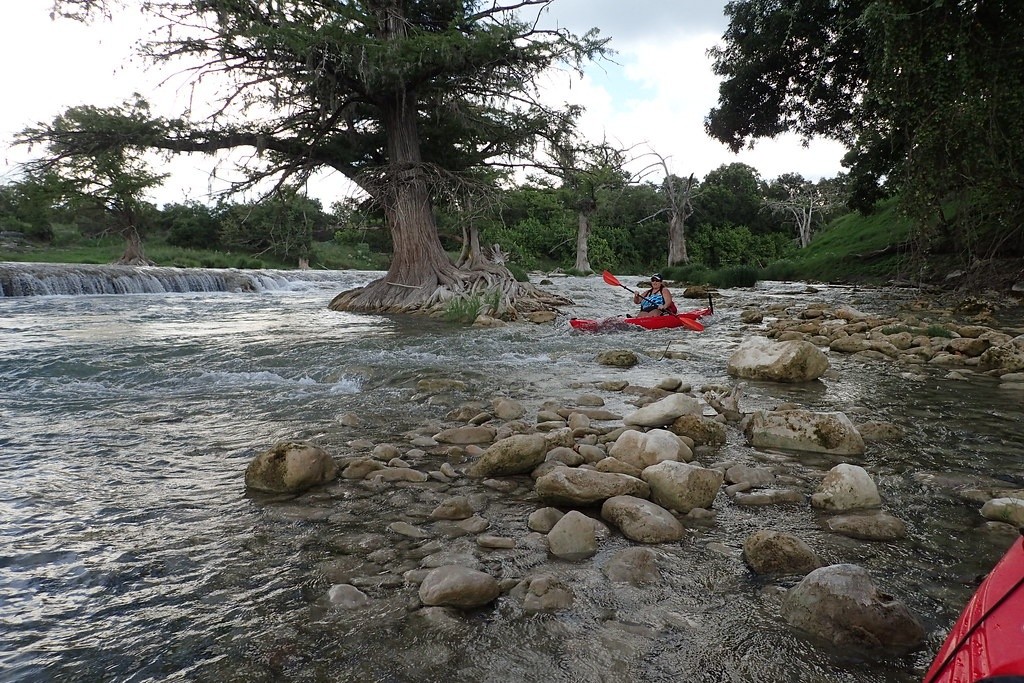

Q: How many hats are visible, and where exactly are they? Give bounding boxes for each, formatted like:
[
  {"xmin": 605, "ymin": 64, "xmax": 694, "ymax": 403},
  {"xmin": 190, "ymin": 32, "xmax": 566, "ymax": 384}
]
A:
[{"xmin": 651, "ymin": 273, "xmax": 663, "ymax": 282}]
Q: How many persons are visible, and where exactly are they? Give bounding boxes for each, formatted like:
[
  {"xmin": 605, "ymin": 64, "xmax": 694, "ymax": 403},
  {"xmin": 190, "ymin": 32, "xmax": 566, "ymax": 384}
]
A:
[{"xmin": 626, "ymin": 273, "xmax": 672, "ymax": 318}]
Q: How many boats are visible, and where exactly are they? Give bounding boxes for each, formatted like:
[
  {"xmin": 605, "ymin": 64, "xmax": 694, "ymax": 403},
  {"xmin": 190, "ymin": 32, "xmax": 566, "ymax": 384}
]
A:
[
  {"xmin": 568, "ymin": 303, "xmax": 712, "ymax": 333},
  {"xmin": 923, "ymin": 529, "xmax": 1024, "ymax": 683}
]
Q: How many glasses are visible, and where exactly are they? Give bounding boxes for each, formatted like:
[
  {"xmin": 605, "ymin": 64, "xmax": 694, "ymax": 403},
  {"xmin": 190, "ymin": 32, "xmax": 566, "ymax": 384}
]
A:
[{"xmin": 651, "ymin": 279, "xmax": 660, "ymax": 282}]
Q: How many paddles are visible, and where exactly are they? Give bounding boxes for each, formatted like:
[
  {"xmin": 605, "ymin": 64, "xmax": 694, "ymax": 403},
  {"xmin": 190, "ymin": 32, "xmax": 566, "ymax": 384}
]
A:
[{"xmin": 602, "ymin": 269, "xmax": 705, "ymax": 332}]
